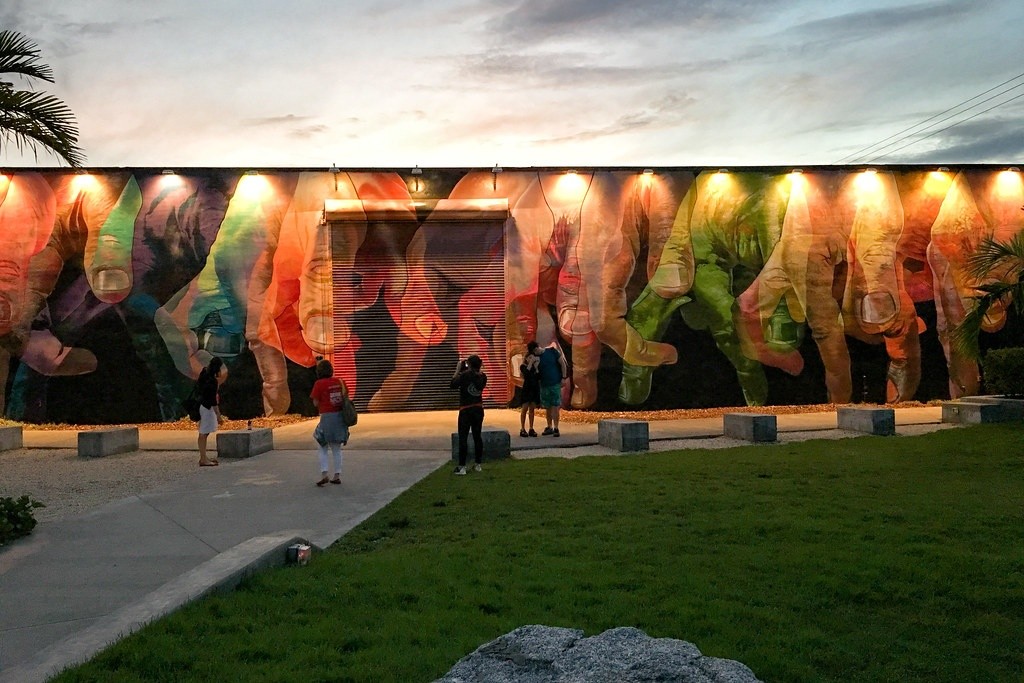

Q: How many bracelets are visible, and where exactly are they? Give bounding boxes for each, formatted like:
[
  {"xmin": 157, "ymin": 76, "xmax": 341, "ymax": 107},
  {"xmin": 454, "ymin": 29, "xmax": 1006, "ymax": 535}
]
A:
[{"xmin": 562, "ymin": 376, "xmax": 568, "ymax": 379}]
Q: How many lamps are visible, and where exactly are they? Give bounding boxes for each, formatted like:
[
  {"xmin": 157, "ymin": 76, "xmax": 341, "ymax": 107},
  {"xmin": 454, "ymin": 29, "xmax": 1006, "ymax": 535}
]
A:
[
  {"xmin": 719, "ymin": 168, "xmax": 729, "ymax": 173},
  {"xmin": 412, "ymin": 165, "xmax": 422, "ymax": 174},
  {"xmin": 244, "ymin": 169, "xmax": 259, "ymax": 175},
  {"xmin": 162, "ymin": 169, "xmax": 174, "ymax": 175},
  {"xmin": 492, "ymin": 164, "xmax": 503, "ymax": 173},
  {"xmin": 865, "ymin": 168, "xmax": 877, "ymax": 173},
  {"xmin": 792, "ymin": 169, "xmax": 803, "ymax": 174},
  {"xmin": 1008, "ymin": 167, "xmax": 1021, "ymax": 172},
  {"xmin": 74, "ymin": 168, "xmax": 88, "ymax": 174},
  {"xmin": 329, "ymin": 163, "xmax": 340, "ymax": 173},
  {"xmin": 936, "ymin": 167, "xmax": 950, "ymax": 172},
  {"xmin": 643, "ymin": 169, "xmax": 654, "ymax": 174},
  {"xmin": 567, "ymin": 169, "xmax": 578, "ymax": 174}
]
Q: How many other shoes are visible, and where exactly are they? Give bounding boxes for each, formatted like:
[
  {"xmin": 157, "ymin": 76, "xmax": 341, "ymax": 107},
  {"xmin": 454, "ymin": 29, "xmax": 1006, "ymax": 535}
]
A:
[
  {"xmin": 316, "ymin": 476, "xmax": 329, "ymax": 485},
  {"xmin": 198, "ymin": 458, "xmax": 218, "ymax": 466},
  {"xmin": 329, "ymin": 478, "xmax": 342, "ymax": 485},
  {"xmin": 454, "ymin": 466, "xmax": 467, "ymax": 475},
  {"xmin": 472, "ymin": 463, "xmax": 482, "ymax": 472}
]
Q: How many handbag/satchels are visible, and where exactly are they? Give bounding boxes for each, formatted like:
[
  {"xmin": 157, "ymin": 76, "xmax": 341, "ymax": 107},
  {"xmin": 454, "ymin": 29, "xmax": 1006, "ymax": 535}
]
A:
[
  {"xmin": 181, "ymin": 397, "xmax": 202, "ymax": 422},
  {"xmin": 338, "ymin": 379, "xmax": 358, "ymax": 427}
]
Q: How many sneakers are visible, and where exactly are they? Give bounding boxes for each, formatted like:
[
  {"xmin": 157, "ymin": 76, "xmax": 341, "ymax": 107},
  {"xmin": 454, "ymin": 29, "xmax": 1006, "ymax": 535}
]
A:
[
  {"xmin": 541, "ymin": 427, "xmax": 553, "ymax": 435},
  {"xmin": 519, "ymin": 429, "xmax": 529, "ymax": 437},
  {"xmin": 552, "ymin": 428, "xmax": 560, "ymax": 437},
  {"xmin": 528, "ymin": 428, "xmax": 538, "ymax": 437}
]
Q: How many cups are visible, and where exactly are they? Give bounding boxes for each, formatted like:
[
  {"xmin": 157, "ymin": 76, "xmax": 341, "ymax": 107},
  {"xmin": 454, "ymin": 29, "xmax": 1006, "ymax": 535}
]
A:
[{"xmin": 248, "ymin": 421, "xmax": 252, "ymax": 430}]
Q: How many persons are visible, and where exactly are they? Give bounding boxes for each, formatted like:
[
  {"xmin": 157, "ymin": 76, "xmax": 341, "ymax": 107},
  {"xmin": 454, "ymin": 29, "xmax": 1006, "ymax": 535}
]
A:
[
  {"xmin": 198, "ymin": 357, "xmax": 223, "ymax": 466},
  {"xmin": 520, "ymin": 352, "xmax": 539, "ymax": 436},
  {"xmin": 527, "ymin": 342, "xmax": 569, "ymax": 436},
  {"xmin": 450, "ymin": 354, "xmax": 487, "ymax": 476},
  {"xmin": 310, "ymin": 360, "xmax": 348, "ymax": 485}
]
